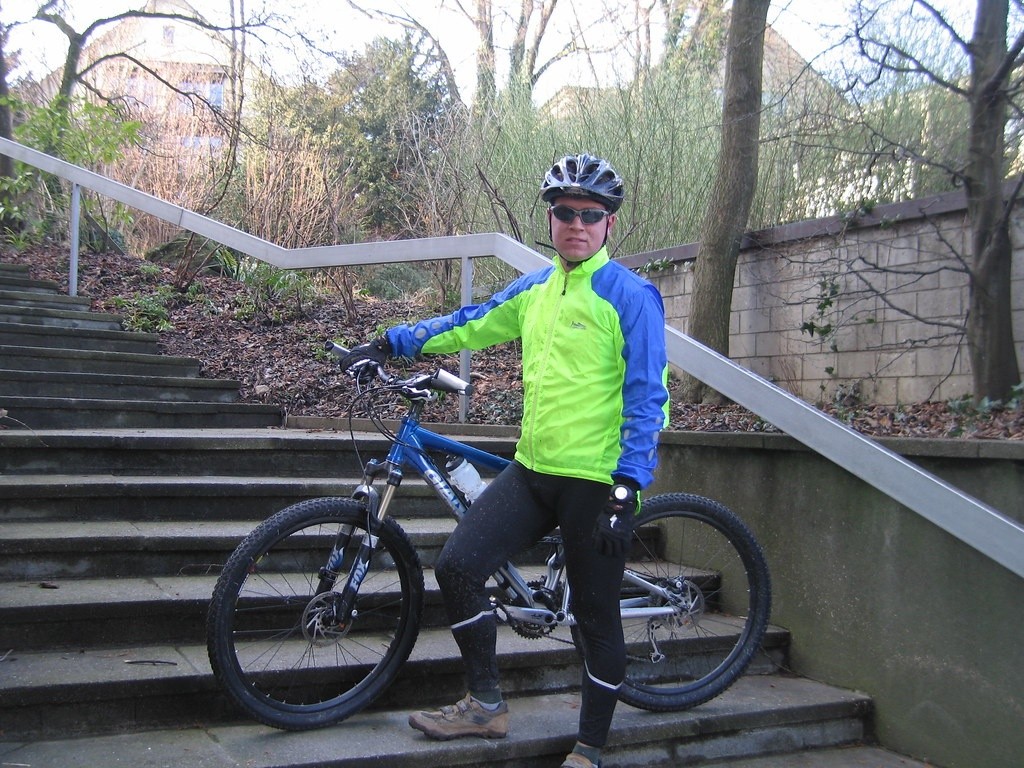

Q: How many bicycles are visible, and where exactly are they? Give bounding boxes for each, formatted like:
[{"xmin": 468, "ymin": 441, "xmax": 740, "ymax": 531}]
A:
[{"xmin": 205, "ymin": 339, "xmax": 774, "ymax": 731}]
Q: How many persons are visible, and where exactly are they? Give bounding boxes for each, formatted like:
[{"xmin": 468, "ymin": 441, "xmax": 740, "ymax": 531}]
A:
[{"xmin": 339, "ymin": 155, "xmax": 671, "ymax": 768}]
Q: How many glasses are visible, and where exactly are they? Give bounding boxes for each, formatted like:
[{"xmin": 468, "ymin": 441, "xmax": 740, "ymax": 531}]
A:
[{"xmin": 549, "ymin": 205, "xmax": 610, "ymax": 225}]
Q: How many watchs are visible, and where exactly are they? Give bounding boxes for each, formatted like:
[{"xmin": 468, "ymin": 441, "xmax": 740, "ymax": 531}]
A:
[{"xmin": 609, "ymin": 484, "xmax": 638, "ymax": 505}]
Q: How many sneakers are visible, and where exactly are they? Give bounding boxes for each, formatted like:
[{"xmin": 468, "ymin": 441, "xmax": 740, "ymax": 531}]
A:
[
  {"xmin": 560, "ymin": 752, "xmax": 602, "ymax": 768},
  {"xmin": 409, "ymin": 693, "xmax": 508, "ymax": 740}
]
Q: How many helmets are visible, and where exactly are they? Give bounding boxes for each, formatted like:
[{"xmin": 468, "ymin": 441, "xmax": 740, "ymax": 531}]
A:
[{"xmin": 539, "ymin": 153, "xmax": 624, "ymax": 216}]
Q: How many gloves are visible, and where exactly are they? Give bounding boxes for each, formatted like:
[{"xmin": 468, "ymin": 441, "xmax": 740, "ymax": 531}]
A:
[
  {"xmin": 590, "ymin": 477, "xmax": 641, "ymax": 557},
  {"xmin": 338, "ymin": 336, "xmax": 390, "ymax": 386}
]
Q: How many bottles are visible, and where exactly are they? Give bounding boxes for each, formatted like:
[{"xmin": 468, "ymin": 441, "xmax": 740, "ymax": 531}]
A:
[{"xmin": 445, "ymin": 454, "xmax": 488, "ymax": 505}]
